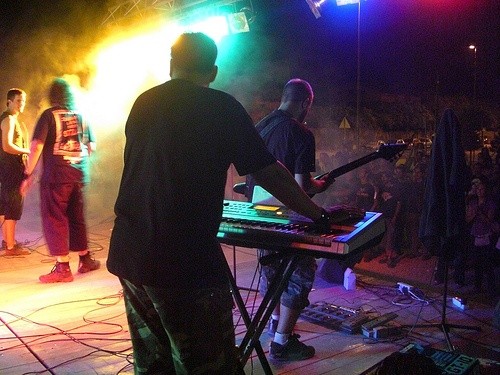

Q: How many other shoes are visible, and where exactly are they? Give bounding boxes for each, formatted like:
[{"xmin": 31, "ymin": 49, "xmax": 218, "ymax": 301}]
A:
[
  {"xmin": 2, "ymin": 239, "xmax": 23, "ymax": 249},
  {"xmin": 267, "ymin": 316, "xmax": 279, "ymax": 336},
  {"xmin": 7, "ymin": 242, "xmax": 31, "ymax": 255},
  {"xmin": 379, "ymin": 256, "xmax": 399, "ymax": 268},
  {"xmin": 268, "ymin": 331, "xmax": 316, "ymax": 362}
]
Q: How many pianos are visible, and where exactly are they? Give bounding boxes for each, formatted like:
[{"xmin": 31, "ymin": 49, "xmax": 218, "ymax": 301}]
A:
[{"xmin": 216, "ymin": 200, "xmax": 390, "ymax": 375}]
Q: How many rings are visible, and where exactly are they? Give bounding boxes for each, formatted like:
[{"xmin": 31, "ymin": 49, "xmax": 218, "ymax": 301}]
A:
[{"xmin": 348, "ymin": 212, "xmax": 352, "ymax": 218}]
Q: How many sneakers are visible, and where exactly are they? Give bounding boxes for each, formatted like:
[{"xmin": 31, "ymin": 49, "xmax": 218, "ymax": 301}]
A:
[
  {"xmin": 78, "ymin": 250, "xmax": 101, "ymax": 273},
  {"xmin": 39, "ymin": 260, "xmax": 73, "ymax": 283}
]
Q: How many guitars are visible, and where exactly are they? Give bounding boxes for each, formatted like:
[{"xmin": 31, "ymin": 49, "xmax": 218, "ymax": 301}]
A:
[{"xmin": 232, "ymin": 137, "xmax": 411, "ymax": 200}]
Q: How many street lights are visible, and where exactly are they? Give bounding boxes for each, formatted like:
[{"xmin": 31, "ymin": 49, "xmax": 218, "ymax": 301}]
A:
[
  {"xmin": 469, "ymin": 45, "xmax": 478, "ymax": 105},
  {"xmin": 306, "ymin": 0, "xmax": 361, "ymax": 142}
]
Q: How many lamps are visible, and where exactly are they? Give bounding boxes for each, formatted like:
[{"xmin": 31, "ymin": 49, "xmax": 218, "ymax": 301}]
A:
[
  {"xmin": 304, "ymin": 0, "xmax": 329, "ymax": 19},
  {"xmin": 228, "ymin": 0, "xmax": 256, "ymax": 34}
]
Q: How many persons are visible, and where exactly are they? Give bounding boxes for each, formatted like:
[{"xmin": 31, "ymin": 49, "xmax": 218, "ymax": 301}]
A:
[
  {"xmin": 246, "ymin": 78, "xmax": 335, "ymax": 364},
  {"xmin": 311, "ymin": 132, "xmax": 500, "ymax": 297},
  {"xmin": 0, "ymin": 88, "xmax": 31, "ymax": 256},
  {"xmin": 107, "ymin": 34, "xmax": 366, "ymax": 375},
  {"xmin": 20, "ymin": 78, "xmax": 100, "ymax": 283}
]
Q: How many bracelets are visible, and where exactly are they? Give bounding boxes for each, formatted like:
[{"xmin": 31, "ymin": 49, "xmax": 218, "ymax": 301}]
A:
[
  {"xmin": 312, "ymin": 208, "xmax": 331, "ymax": 225},
  {"xmin": 24, "ymin": 170, "xmax": 32, "ymax": 177}
]
